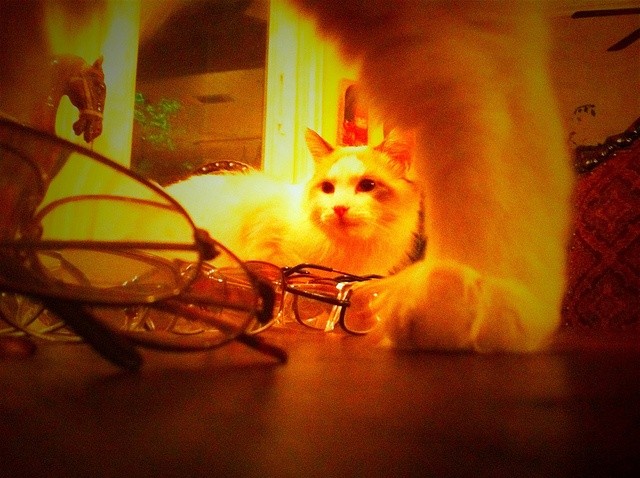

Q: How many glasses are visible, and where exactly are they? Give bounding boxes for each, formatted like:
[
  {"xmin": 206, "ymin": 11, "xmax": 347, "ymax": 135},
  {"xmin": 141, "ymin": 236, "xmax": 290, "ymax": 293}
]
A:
[{"xmin": 0, "ymin": 113, "xmax": 386, "ymax": 369}]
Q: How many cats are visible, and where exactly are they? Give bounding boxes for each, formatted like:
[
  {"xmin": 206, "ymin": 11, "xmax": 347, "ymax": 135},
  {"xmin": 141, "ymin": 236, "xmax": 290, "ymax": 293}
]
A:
[
  {"xmin": 125, "ymin": 124, "xmax": 423, "ymax": 277},
  {"xmin": 1, "ymin": 0, "xmax": 574, "ymax": 356}
]
